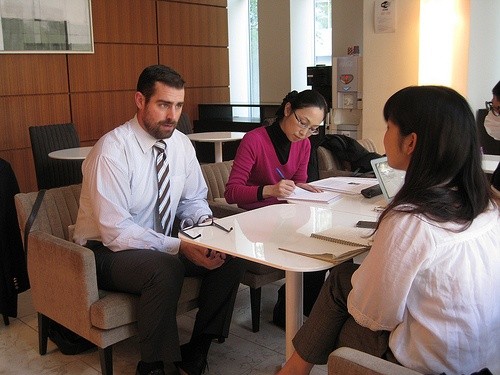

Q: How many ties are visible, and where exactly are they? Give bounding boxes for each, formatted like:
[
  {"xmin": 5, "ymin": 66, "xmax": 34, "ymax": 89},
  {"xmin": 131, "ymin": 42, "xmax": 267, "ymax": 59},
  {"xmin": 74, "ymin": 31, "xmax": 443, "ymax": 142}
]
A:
[{"xmin": 153, "ymin": 140, "xmax": 171, "ymax": 238}]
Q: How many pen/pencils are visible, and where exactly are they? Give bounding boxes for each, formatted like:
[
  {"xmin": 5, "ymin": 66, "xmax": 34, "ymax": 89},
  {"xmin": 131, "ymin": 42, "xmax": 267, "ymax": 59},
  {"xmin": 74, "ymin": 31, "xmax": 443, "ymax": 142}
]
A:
[
  {"xmin": 276, "ymin": 168, "xmax": 296, "ymax": 195},
  {"xmin": 480, "ymin": 147, "xmax": 483, "ymax": 156}
]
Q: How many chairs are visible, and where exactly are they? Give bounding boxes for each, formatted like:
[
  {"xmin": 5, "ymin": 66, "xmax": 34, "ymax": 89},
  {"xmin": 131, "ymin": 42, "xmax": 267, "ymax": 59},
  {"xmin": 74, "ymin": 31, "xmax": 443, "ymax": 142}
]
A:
[
  {"xmin": 28, "ymin": 122, "xmax": 83, "ymax": 189},
  {"xmin": 200, "ymin": 161, "xmax": 285, "ymax": 332},
  {"xmin": 315, "ymin": 145, "xmax": 376, "ymax": 178},
  {"xmin": 176, "ymin": 113, "xmax": 196, "ymax": 147},
  {"xmin": 14, "ymin": 184, "xmax": 248, "ymax": 375}
]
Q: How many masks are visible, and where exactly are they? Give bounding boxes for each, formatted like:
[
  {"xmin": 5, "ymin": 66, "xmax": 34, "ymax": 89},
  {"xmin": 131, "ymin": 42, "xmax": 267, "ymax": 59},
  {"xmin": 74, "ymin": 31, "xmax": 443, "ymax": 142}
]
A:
[{"xmin": 484, "ymin": 111, "xmax": 500, "ymax": 141}]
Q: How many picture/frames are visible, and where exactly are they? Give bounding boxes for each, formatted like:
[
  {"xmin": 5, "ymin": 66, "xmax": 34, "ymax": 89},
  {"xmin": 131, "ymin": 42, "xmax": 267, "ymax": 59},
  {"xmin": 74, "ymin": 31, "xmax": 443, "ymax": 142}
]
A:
[{"xmin": 0, "ymin": 0, "xmax": 95, "ymax": 55}]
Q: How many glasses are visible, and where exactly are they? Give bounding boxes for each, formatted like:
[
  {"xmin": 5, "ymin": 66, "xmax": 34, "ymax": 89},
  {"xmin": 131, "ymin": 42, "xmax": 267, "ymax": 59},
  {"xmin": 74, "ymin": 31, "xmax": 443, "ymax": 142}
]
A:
[
  {"xmin": 178, "ymin": 214, "xmax": 234, "ymax": 239},
  {"xmin": 485, "ymin": 101, "xmax": 500, "ymax": 117},
  {"xmin": 291, "ymin": 107, "xmax": 320, "ymax": 136}
]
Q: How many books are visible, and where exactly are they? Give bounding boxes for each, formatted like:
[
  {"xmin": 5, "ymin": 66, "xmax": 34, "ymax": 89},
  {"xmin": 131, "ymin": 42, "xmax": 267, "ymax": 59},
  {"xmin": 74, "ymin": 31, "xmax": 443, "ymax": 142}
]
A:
[{"xmin": 278, "ymin": 226, "xmax": 376, "ymax": 265}]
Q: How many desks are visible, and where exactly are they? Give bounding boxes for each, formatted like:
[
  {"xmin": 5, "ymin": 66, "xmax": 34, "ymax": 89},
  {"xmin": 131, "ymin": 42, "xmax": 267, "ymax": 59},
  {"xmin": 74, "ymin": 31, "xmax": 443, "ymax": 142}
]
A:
[
  {"xmin": 288, "ymin": 194, "xmax": 388, "ymax": 218},
  {"xmin": 178, "ymin": 203, "xmax": 377, "ymax": 364},
  {"xmin": 48, "ymin": 147, "xmax": 92, "ymax": 159},
  {"xmin": 185, "ymin": 131, "xmax": 247, "ymax": 163}
]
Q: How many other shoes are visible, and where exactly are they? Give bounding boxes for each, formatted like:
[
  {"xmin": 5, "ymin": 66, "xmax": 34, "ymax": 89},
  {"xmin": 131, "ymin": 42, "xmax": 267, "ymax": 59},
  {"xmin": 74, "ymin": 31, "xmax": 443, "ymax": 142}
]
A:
[
  {"xmin": 172, "ymin": 345, "xmax": 209, "ymax": 375},
  {"xmin": 135, "ymin": 360, "xmax": 166, "ymax": 375},
  {"xmin": 273, "ymin": 309, "xmax": 287, "ymax": 330}
]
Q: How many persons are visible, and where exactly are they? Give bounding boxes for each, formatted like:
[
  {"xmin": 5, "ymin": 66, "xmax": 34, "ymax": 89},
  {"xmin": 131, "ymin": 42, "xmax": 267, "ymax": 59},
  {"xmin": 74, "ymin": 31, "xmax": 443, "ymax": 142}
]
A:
[
  {"xmin": 73, "ymin": 64, "xmax": 235, "ymax": 375},
  {"xmin": 223, "ymin": 89, "xmax": 328, "ymax": 332},
  {"xmin": 277, "ymin": 85, "xmax": 500, "ymax": 375},
  {"xmin": 476, "ymin": 81, "xmax": 500, "ymax": 200}
]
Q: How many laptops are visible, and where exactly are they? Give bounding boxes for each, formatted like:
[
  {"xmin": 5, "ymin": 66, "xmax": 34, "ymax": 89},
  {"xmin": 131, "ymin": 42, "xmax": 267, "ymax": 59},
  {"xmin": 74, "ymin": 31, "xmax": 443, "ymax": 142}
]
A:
[{"xmin": 370, "ymin": 157, "xmax": 407, "ymax": 204}]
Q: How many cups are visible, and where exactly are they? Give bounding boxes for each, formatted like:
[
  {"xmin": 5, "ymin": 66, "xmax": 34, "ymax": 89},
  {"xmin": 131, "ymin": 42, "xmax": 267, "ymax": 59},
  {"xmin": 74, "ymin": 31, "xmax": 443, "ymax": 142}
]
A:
[
  {"xmin": 346, "ymin": 47, "xmax": 352, "ymax": 56},
  {"xmin": 352, "ymin": 46, "xmax": 360, "ymax": 54}
]
